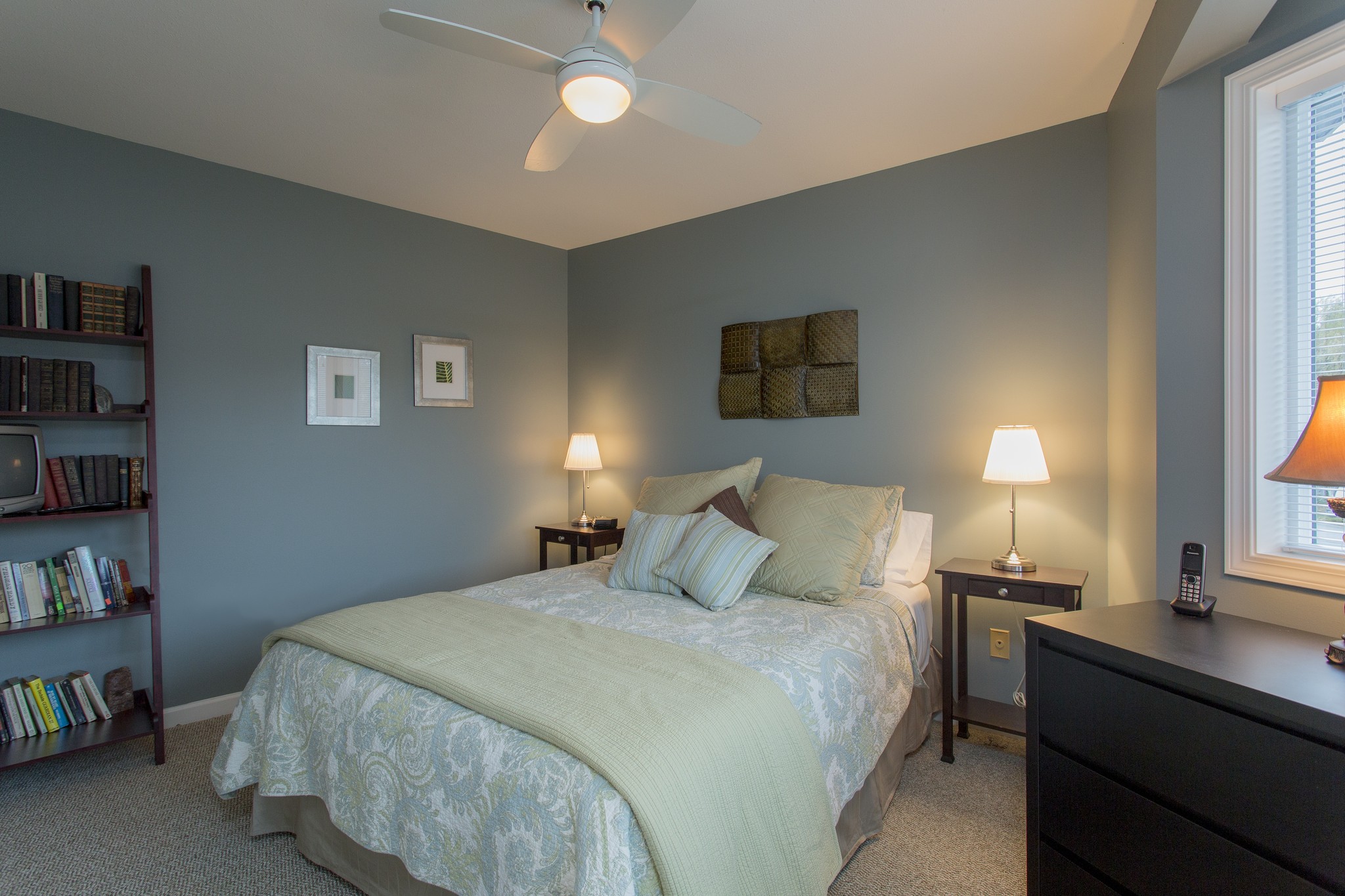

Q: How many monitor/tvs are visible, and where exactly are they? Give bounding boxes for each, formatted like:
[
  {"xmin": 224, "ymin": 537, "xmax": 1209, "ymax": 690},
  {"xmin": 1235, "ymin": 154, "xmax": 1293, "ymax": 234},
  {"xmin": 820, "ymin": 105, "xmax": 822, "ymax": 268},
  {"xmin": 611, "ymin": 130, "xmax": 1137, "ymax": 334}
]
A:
[{"xmin": 0, "ymin": 423, "xmax": 46, "ymax": 519}]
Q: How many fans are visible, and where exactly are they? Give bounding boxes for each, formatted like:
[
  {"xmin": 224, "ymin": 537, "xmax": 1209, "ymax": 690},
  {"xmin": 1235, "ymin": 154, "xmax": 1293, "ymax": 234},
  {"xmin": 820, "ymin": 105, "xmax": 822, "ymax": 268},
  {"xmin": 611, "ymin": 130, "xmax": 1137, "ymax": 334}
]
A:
[{"xmin": 372, "ymin": 0, "xmax": 764, "ymax": 172}]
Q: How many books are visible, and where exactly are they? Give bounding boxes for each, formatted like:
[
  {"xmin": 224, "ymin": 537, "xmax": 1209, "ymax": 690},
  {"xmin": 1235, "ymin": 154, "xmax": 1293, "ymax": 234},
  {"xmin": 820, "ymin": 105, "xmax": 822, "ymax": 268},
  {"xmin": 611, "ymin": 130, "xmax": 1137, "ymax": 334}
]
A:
[{"xmin": 0, "ymin": 269, "xmax": 149, "ymax": 744}]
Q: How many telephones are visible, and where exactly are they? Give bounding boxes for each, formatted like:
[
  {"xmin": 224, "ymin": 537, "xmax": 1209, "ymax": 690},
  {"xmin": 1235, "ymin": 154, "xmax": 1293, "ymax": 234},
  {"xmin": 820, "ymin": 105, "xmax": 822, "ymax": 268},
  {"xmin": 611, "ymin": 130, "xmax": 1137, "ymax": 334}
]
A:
[{"xmin": 1170, "ymin": 542, "xmax": 1217, "ymax": 618}]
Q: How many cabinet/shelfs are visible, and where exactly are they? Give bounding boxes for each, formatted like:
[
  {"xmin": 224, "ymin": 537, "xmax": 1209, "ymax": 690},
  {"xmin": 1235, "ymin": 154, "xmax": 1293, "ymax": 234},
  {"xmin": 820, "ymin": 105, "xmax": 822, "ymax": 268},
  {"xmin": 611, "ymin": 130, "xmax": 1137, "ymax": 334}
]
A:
[
  {"xmin": 1, "ymin": 262, "xmax": 167, "ymax": 771},
  {"xmin": 1021, "ymin": 599, "xmax": 1345, "ymax": 896}
]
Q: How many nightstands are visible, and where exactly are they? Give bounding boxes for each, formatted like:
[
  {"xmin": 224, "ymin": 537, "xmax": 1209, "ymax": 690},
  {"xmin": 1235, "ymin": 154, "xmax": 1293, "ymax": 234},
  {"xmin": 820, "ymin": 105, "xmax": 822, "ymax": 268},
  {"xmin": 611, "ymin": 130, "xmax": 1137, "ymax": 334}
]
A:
[
  {"xmin": 933, "ymin": 557, "xmax": 1089, "ymax": 765},
  {"xmin": 535, "ymin": 521, "xmax": 626, "ymax": 571}
]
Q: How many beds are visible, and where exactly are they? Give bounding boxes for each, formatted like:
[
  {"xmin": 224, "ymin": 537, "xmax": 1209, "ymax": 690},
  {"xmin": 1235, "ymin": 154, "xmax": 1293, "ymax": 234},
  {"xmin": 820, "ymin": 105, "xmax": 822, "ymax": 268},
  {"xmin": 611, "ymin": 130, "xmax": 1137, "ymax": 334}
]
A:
[{"xmin": 249, "ymin": 552, "xmax": 945, "ymax": 896}]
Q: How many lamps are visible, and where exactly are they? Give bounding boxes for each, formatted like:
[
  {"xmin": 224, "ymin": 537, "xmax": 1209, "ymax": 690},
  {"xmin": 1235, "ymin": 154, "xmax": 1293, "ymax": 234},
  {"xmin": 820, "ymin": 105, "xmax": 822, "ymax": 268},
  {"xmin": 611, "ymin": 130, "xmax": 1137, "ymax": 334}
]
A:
[
  {"xmin": 563, "ymin": 433, "xmax": 602, "ymax": 526},
  {"xmin": 1262, "ymin": 375, "xmax": 1345, "ymax": 668},
  {"xmin": 981, "ymin": 424, "xmax": 1051, "ymax": 573}
]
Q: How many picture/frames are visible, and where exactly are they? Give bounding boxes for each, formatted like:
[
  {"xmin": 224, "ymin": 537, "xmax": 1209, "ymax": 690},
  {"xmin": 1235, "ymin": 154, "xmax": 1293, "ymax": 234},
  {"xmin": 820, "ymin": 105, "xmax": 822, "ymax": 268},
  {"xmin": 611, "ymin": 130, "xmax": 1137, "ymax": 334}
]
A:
[
  {"xmin": 413, "ymin": 334, "xmax": 474, "ymax": 408},
  {"xmin": 306, "ymin": 345, "xmax": 381, "ymax": 427}
]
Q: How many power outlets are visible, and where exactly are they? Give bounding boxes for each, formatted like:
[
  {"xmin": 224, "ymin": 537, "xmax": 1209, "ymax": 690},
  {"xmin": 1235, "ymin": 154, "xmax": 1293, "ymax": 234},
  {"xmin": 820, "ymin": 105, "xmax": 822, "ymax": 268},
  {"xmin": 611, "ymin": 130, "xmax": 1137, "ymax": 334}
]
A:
[{"xmin": 988, "ymin": 628, "xmax": 1012, "ymax": 661}]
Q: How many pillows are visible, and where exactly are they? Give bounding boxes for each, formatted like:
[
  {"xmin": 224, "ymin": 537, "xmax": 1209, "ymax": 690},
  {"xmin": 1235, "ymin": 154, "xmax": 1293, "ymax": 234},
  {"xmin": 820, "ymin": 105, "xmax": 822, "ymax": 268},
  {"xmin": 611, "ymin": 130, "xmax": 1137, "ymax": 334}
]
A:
[
  {"xmin": 605, "ymin": 508, "xmax": 706, "ymax": 597},
  {"xmin": 681, "ymin": 485, "xmax": 772, "ymax": 595},
  {"xmin": 615, "ymin": 457, "xmax": 764, "ymax": 561},
  {"xmin": 652, "ymin": 504, "xmax": 779, "ymax": 612},
  {"xmin": 859, "ymin": 492, "xmax": 903, "ymax": 586},
  {"xmin": 884, "ymin": 510, "xmax": 934, "ymax": 585},
  {"xmin": 745, "ymin": 472, "xmax": 905, "ymax": 607}
]
